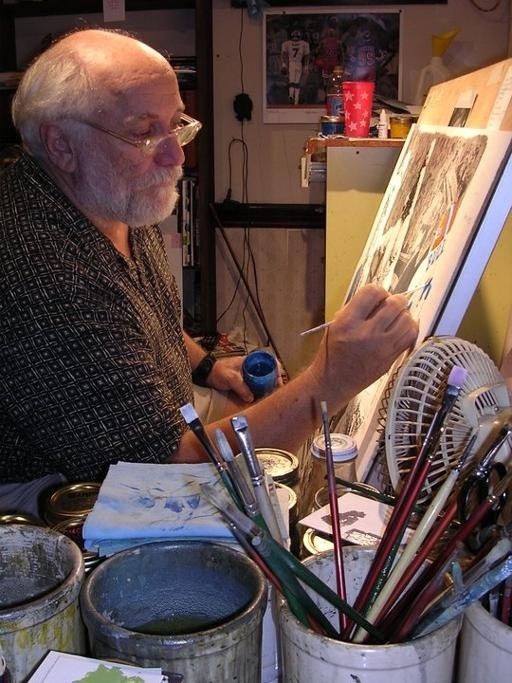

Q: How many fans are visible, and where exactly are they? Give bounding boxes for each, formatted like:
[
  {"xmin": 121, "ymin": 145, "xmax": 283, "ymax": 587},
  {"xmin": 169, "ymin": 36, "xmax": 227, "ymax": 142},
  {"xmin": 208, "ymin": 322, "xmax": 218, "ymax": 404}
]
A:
[{"xmin": 373, "ymin": 333, "xmax": 512, "ymax": 519}]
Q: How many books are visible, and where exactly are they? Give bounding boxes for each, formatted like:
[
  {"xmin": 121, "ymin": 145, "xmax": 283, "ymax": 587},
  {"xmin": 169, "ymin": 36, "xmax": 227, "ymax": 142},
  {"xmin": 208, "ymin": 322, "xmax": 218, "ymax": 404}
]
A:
[{"xmin": 82, "ymin": 459, "xmax": 270, "ymax": 555}]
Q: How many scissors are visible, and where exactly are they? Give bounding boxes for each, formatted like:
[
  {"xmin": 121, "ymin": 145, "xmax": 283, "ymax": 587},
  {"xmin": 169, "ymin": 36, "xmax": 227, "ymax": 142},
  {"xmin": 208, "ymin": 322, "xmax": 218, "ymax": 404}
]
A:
[{"xmin": 457, "ymin": 460, "xmax": 509, "ymax": 556}]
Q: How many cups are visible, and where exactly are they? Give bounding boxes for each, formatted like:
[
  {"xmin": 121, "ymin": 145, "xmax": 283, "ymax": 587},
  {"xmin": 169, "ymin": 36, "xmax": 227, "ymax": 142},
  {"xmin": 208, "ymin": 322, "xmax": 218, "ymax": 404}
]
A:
[
  {"xmin": 82, "ymin": 539, "xmax": 269, "ymax": 682},
  {"xmin": 459, "ymin": 590, "xmax": 512, "ymax": 683},
  {"xmin": 271, "ymin": 544, "xmax": 461, "ymax": 679},
  {"xmin": 1, "ymin": 521, "xmax": 85, "ymax": 682},
  {"xmin": 342, "ymin": 80, "xmax": 376, "ymax": 138},
  {"xmin": 240, "ymin": 349, "xmax": 279, "ymax": 398}
]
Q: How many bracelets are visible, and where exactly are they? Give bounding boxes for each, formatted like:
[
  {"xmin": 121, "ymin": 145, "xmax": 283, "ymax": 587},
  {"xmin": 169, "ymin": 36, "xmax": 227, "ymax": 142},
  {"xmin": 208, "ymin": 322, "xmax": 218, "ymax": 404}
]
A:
[{"xmin": 192, "ymin": 354, "xmax": 217, "ymax": 386}]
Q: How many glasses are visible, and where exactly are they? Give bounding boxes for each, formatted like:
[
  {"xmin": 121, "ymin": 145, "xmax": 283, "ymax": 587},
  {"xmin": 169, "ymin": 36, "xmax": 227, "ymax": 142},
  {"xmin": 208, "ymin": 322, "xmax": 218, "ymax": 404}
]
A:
[{"xmin": 54, "ymin": 113, "xmax": 203, "ymax": 153}]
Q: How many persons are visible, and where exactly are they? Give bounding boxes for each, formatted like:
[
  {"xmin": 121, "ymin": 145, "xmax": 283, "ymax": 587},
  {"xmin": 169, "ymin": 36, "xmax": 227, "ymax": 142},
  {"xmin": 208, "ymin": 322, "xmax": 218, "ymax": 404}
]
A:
[
  {"xmin": 1, "ymin": 28, "xmax": 418, "ymax": 521},
  {"xmin": 265, "ymin": 13, "xmax": 398, "ymax": 105}
]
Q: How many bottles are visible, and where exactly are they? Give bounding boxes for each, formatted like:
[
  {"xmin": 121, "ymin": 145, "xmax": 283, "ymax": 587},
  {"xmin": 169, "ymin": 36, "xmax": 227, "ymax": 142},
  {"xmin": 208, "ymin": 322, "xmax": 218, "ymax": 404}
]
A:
[
  {"xmin": 229, "ymin": 445, "xmax": 298, "ymax": 550},
  {"xmin": 326, "ymin": 66, "xmax": 346, "ymax": 117},
  {"xmin": 298, "ymin": 433, "xmax": 360, "ymax": 560}
]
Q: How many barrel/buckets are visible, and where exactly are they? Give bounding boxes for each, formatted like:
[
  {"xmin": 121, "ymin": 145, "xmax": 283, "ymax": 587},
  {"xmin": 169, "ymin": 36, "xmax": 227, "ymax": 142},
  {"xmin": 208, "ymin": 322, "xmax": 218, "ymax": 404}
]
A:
[
  {"xmin": 443, "ymin": 39, "xmax": 481, "ymax": 65},
  {"xmin": 414, "ymin": 56, "xmax": 451, "ymax": 106}
]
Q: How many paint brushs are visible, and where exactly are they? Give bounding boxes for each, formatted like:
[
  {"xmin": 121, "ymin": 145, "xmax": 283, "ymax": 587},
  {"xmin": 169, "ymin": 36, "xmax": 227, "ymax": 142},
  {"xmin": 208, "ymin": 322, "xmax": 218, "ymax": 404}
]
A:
[
  {"xmin": 299, "ymin": 284, "xmax": 429, "ymax": 338},
  {"xmin": 175, "ymin": 365, "xmax": 512, "ymax": 641}
]
